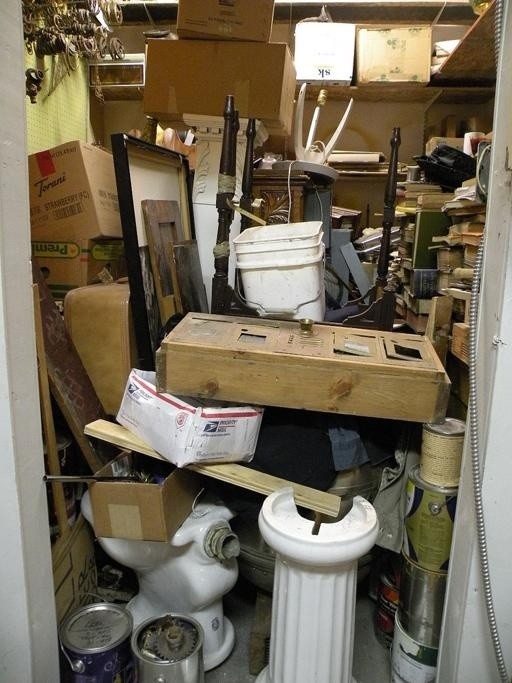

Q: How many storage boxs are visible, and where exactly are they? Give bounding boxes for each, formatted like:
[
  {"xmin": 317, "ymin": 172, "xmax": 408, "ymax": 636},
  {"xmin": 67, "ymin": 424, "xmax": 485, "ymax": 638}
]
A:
[
  {"xmin": 294, "ymin": 21, "xmax": 358, "ymax": 84},
  {"xmin": 88, "ymin": 450, "xmax": 205, "ymax": 544},
  {"xmin": 113, "ymin": 368, "xmax": 265, "ymax": 471},
  {"xmin": 359, "ymin": 25, "xmax": 433, "ymax": 85},
  {"xmin": 31, "ymin": 243, "xmax": 125, "ymax": 305},
  {"xmin": 174, "ymin": 0, "xmax": 277, "ymax": 48},
  {"xmin": 30, "ymin": 141, "xmax": 123, "ymax": 240},
  {"xmin": 143, "ymin": 39, "xmax": 295, "ymax": 136}
]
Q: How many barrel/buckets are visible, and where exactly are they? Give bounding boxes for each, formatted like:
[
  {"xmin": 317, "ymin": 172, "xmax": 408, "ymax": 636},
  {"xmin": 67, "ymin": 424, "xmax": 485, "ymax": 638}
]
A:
[
  {"xmin": 388, "ymin": 608, "xmax": 439, "ymax": 683},
  {"xmin": 395, "ymin": 546, "xmax": 448, "ymax": 650},
  {"xmin": 56, "ymin": 590, "xmax": 134, "ymax": 682},
  {"xmin": 232, "ymin": 217, "xmax": 329, "ymax": 322},
  {"xmin": 401, "ymin": 462, "xmax": 460, "ymax": 573}
]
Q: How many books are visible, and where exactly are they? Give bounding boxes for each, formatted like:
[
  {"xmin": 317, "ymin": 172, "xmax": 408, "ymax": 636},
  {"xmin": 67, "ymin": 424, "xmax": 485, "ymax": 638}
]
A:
[{"xmin": 386, "ymin": 180, "xmax": 486, "ymax": 290}]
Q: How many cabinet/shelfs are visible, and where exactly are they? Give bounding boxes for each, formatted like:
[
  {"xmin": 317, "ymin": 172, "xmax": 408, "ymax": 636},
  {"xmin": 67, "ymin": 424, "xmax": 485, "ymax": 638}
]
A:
[
  {"xmin": 34, "ymin": 284, "xmax": 99, "ymax": 638},
  {"xmin": 291, "ymin": 2, "xmax": 508, "ymax": 340}
]
